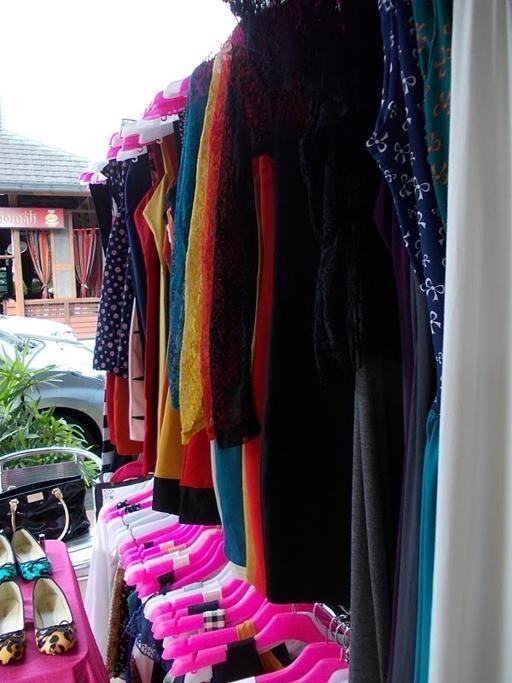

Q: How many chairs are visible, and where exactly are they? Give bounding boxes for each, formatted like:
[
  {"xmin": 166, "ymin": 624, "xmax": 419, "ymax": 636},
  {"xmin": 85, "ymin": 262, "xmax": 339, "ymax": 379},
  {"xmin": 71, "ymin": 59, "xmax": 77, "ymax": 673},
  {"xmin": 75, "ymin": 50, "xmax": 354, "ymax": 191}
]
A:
[{"xmin": 0, "ymin": 446, "xmax": 102, "ymax": 616}]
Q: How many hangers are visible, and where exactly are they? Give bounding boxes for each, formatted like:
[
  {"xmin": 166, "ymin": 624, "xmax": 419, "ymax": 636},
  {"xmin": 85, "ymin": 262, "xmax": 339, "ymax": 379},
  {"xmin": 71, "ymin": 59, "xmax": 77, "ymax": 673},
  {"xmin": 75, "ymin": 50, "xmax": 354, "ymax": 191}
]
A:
[
  {"xmin": 90, "ymin": 458, "xmax": 351, "ymax": 682},
  {"xmin": 75, "ymin": 51, "xmax": 208, "ymax": 186}
]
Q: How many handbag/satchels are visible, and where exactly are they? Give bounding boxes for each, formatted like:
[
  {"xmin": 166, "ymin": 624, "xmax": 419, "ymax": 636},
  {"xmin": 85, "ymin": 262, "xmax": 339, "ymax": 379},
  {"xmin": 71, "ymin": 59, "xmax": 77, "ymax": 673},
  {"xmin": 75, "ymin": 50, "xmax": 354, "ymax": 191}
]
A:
[{"xmin": 0, "ymin": 446, "xmax": 103, "ymax": 542}]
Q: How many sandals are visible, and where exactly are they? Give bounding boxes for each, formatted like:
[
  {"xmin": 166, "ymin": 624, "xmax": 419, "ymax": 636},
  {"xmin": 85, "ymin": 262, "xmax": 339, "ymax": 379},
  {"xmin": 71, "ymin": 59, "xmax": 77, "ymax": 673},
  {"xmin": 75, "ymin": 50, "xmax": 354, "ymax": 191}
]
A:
[
  {"xmin": 12, "ymin": 526, "xmax": 52, "ymax": 580},
  {"xmin": 0, "ymin": 580, "xmax": 26, "ymax": 665},
  {"xmin": 31, "ymin": 576, "xmax": 78, "ymax": 655},
  {"xmin": 0, "ymin": 534, "xmax": 16, "ymax": 582}
]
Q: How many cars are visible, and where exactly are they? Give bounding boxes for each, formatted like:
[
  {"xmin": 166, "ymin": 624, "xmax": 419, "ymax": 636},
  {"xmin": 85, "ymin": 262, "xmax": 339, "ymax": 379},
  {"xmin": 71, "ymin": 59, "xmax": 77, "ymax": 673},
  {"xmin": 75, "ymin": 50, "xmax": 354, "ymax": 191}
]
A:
[
  {"xmin": 0, "ymin": 311, "xmax": 78, "ymax": 342},
  {"xmin": 0, "ymin": 326, "xmax": 107, "ymax": 462}
]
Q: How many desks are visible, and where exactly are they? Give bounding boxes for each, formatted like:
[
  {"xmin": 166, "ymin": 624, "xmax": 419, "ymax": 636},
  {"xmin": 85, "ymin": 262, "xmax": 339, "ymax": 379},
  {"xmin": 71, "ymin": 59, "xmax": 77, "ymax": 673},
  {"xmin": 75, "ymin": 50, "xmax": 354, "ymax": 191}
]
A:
[{"xmin": 0, "ymin": 532, "xmax": 109, "ymax": 683}]
